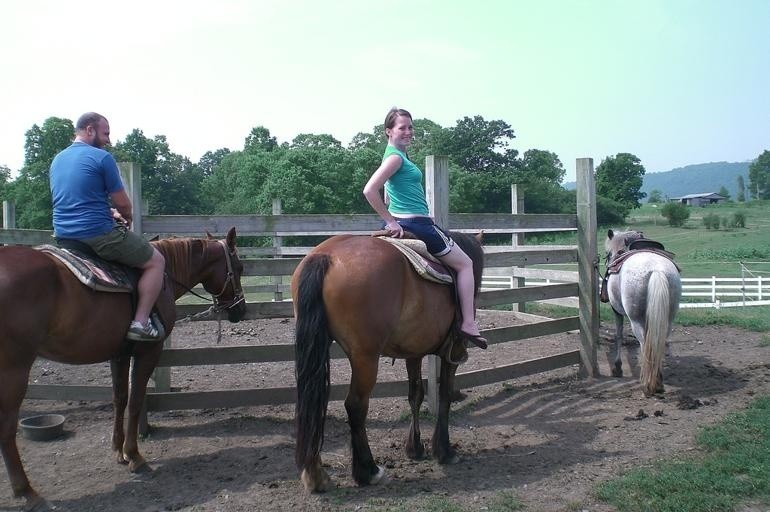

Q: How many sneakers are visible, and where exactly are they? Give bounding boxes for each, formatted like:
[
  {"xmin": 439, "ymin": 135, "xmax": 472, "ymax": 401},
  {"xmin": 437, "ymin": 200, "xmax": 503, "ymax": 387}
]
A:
[{"xmin": 127, "ymin": 318, "xmax": 162, "ymax": 343}]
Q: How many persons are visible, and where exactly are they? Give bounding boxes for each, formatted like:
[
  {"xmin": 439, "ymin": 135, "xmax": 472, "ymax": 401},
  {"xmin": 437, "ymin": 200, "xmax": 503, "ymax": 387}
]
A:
[
  {"xmin": 362, "ymin": 107, "xmax": 491, "ymax": 350},
  {"xmin": 49, "ymin": 112, "xmax": 167, "ymax": 343}
]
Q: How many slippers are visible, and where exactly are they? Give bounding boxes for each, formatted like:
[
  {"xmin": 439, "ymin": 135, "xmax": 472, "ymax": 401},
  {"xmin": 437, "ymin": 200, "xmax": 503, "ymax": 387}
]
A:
[{"xmin": 460, "ymin": 327, "xmax": 488, "ymax": 351}]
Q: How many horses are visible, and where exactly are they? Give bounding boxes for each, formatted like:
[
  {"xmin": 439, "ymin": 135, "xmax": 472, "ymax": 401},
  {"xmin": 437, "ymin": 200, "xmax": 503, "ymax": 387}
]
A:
[
  {"xmin": 288, "ymin": 225, "xmax": 488, "ymax": 493},
  {"xmin": 602, "ymin": 229, "xmax": 682, "ymax": 398},
  {"xmin": 0, "ymin": 226, "xmax": 250, "ymax": 512}
]
